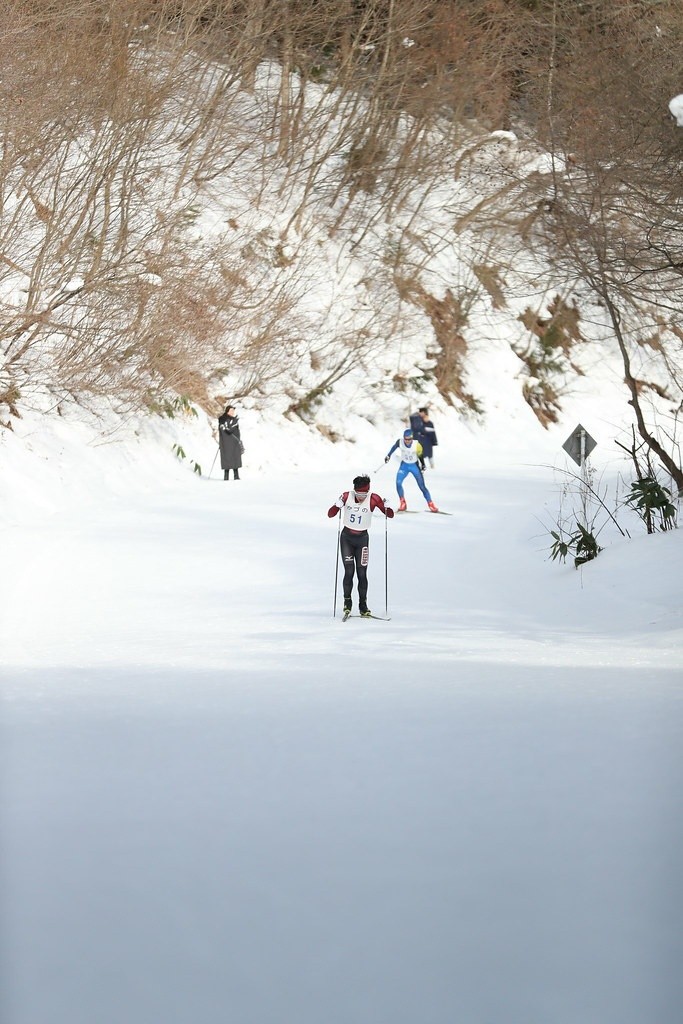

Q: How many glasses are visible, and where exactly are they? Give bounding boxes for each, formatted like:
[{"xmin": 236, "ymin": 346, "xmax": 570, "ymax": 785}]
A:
[
  {"xmin": 352, "ymin": 490, "xmax": 368, "ymax": 498},
  {"xmin": 404, "ymin": 438, "xmax": 412, "ymax": 440}
]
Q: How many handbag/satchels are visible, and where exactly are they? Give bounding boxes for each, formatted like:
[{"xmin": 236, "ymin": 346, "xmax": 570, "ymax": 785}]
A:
[{"xmin": 239, "ymin": 440, "xmax": 245, "ymax": 455}]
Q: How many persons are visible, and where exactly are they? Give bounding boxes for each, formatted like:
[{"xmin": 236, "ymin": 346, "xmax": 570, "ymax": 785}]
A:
[
  {"xmin": 218, "ymin": 406, "xmax": 244, "ymax": 481},
  {"xmin": 409, "ymin": 408, "xmax": 439, "ymax": 469},
  {"xmin": 384, "ymin": 429, "xmax": 438, "ymax": 513},
  {"xmin": 328, "ymin": 476, "xmax": 394, "ymax": 617}
]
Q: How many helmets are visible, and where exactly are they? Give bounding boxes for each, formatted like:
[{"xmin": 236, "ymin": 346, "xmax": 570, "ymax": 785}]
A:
[{"xmin": 404, "ymin": 429, "xmax": 414, "ymax": 437}]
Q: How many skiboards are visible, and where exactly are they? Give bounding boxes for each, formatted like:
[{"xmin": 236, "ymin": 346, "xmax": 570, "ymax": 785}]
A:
[
  {"xmin": 392, "ymin": 508, "xmax": 457, "ymax": 516},
  {"xmin": 338, "ymin": 610, "xmax": 395, "ymax": 622}
]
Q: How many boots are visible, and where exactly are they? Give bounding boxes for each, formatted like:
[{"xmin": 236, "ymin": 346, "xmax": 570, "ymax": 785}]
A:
[
  {"xmin": 233, "ymin": 468, "xmax": 240, "ymax": 480},
  {"xmin": 224, "ymin": 470, "xmax": 229, "ymax": 480}
]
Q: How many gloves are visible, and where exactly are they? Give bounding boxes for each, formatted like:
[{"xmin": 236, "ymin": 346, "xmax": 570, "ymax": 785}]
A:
[
  {"xmin": 384, "ymin": 507, "xmax": 394, "ymax": 518},
  {"xmin": 228, "ymin": 414, "xmax": 239, "ymax": 428},
  {"xmin": 385, "ymin": 455, "xmax": 390, "ymax": 463},
  {"xmin": 335, "ymin": 495, "xmax": 345, "ymax": 508},
  {"xmin": 420, "ymin": 465, "xmax": 426, "ymax": 473}
]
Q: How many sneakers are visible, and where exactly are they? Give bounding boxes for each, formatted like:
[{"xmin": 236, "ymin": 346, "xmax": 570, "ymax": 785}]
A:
[
  {"xmin": 398, "ymin": 498, "xmax": 407, "ymax": 511},
  {"xmin": 428, "ymin": 501, "xmax": 439, "ymax": 513},
  {"xmin": 343, "ymin": 599, "xmax": 352, "ymax": 614},
  {"xmin": 359, "ymin": 602, "xmax": 371, "ymax": 616}
]
